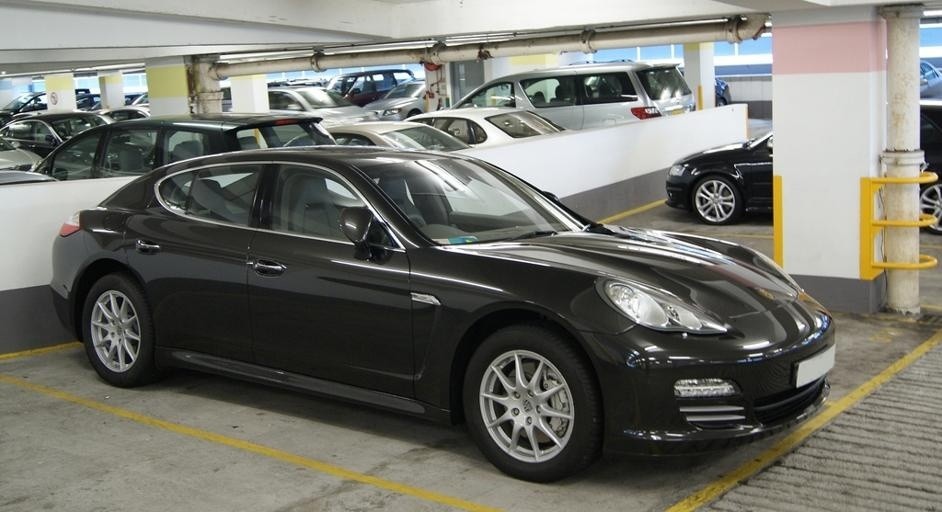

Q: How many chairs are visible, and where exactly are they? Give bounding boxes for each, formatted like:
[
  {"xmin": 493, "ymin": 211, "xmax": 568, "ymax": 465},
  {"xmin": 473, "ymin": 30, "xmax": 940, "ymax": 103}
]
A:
[
  {"xmin": 288, "ymin": 176, "xmax": 341, "ymax": 239},
  {"xmin": 532, "ymin": 82, "xmax": 619, "ymax": 105},
  {"xmin": 188, "ymin": 179, "xmax": 232, "ymax": 222},
  {"xmin": 171, "ymin": 141, "xmax": 203, "ymax": 163},
  {"xmin": 378, "ymin": 171, "xmax": 426, "ymax": 227},
  {"xmin": 118, "ymin": 147, "xmax": 151, "ymax": 173}
]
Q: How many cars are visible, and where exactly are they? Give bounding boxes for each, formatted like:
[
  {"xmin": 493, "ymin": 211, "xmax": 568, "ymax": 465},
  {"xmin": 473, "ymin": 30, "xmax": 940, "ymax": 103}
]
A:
[
  {"xmin": 666, "ymin": 102, "xmax": 941, "ymax": 237},
  {"xmin": 48, "ymin": 142, "xmax": 838, "ymax": 485},
  {"xmin": 919, "ymin": 60, "xmax": 941, "ymax": 100}
]
